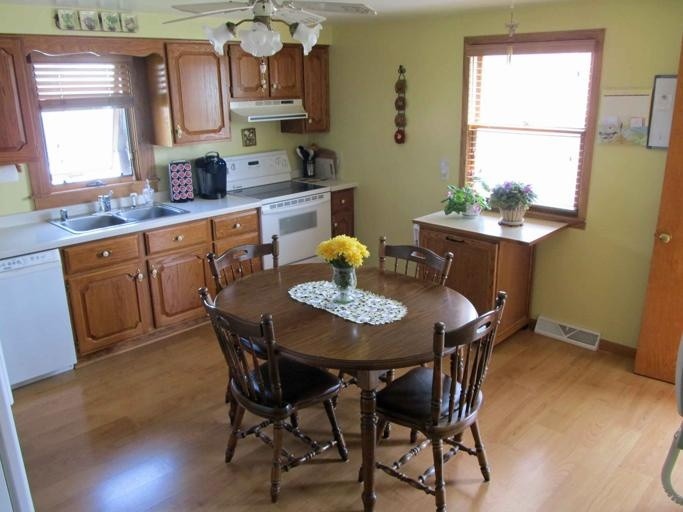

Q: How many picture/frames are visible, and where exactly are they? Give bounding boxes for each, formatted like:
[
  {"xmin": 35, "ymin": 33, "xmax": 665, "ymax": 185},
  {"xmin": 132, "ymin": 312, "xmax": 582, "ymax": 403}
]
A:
[{"xmin": 646, "ymin": 73, "xmax": 677, "ymax": 151}]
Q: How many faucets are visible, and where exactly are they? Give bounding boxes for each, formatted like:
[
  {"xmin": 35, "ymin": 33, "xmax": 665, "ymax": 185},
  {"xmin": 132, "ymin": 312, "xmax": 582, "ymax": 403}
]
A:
[
  {"xmin": 59, "ymin": 208, "xmax": 72, "ymax": 226},
  {"xmin": 130, "ymin": 192, "xmax": 138, "ymax": 209}
]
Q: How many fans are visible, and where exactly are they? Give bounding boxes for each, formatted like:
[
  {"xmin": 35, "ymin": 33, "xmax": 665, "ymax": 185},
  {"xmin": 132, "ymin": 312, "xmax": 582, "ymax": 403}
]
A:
[{"xmin": 163, "ymin": 0, "xmax": 379, "ymax": 26}]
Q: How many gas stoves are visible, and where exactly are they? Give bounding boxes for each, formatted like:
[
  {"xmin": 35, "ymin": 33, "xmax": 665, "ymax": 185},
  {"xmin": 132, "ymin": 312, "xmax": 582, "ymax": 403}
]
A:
[{"xmin": 226, "ymin": 150, "xmax": 331, "ymax": 204}]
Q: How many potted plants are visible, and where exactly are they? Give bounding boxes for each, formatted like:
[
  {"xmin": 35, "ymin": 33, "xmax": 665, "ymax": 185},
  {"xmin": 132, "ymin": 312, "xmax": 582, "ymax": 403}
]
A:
[
  {"xmin": 441, "ymin": 183, "xmax": 486, "ymax": 219},
  {"xmin": 487, "ymin": 181, "xmax": 537, "ymax": 227}
]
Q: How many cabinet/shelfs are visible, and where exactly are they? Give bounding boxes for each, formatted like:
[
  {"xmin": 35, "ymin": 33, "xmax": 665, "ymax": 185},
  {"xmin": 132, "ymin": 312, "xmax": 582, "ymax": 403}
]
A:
[
  {"xmin": 228, "ymin": 43, "xmax": 304, "ymax": 102},
  {"xmin": 146, "ymin": 40, "xmax": 232, "ymax": 149},
  {"xmin": 0, "ymin": 35, "xmax": 46, "ymax": 164},
  {"xmin": 411, "ymin": 207, "xmax": 569, "ymax": 351},
  {"xmin": 331, "ymin": 188, "xmax": 355, "ymax": 238},
  {"xmin": 62, "ymin": 220, "xmax": 218, "ymax": 357},
  {"xmin": 281, "ymin": 46, "xmax": 331, "ymax": 135},
  {"xmin": 213, "ymin": 211, "xmax": 264, "ymax": 292}
]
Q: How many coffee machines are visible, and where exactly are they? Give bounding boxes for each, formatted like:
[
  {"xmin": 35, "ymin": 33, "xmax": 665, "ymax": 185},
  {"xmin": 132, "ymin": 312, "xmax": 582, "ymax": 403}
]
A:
[{"xmin": 195, "ymin": 155, "xmax": 227, "ymax": 200}]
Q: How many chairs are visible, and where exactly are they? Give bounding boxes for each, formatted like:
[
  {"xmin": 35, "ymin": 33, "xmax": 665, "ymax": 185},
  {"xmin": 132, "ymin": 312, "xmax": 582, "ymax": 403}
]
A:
[
  {"xmin": 330, "ymin": 234, "xmax": 455, "ymax": 439},
  {"xmin": 357, "ymin": 290, "xmax": 509, "ymax": 512},
  {"xmin": 197, "ymin": 287, "xmax": 350, "ymax": 501},
  {"xmin": 207, "ymin": 235, "xmax": 280, "ymax": 427}
]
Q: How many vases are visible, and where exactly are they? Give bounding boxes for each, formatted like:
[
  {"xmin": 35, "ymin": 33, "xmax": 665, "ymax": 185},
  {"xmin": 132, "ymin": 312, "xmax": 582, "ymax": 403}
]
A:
[{"xmin": 329, "ymin": 266, "xmax": 357, "ymax": 304}]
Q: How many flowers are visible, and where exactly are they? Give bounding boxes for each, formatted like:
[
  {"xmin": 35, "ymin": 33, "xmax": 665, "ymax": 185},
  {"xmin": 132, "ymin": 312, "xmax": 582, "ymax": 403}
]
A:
[{"xmin": 315, "ymin": 235, "xmax": 371, "ymax": 294}]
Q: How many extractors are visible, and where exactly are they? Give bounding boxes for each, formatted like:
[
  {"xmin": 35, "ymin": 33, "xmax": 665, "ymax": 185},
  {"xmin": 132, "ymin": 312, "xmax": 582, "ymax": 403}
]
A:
[{"xmin": 230, "ymin": 99, "xmax": 310, "ymax": 124}]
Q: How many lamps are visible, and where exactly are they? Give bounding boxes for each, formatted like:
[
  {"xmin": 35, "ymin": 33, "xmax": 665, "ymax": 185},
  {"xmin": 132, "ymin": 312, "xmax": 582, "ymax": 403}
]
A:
[{"xmin": 201, "ymin": 10, "xmax": 326, "ymax": 59}]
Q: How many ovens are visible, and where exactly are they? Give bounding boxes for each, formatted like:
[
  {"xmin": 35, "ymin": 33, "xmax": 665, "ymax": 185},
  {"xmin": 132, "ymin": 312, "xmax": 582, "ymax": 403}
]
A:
[{"xmin": 261, "ymin": 193, "xmax": 332, "ymax": 273}]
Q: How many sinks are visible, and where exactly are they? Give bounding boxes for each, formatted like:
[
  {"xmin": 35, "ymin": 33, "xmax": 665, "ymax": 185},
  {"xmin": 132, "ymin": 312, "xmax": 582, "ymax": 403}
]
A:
[
  {"xmin": 116, "ymin": 203, "xmax": 192, "ymax": 224},
  {"xmin": 47, "ymin": 201, "xmax": 128, "ymax": 235}
]
